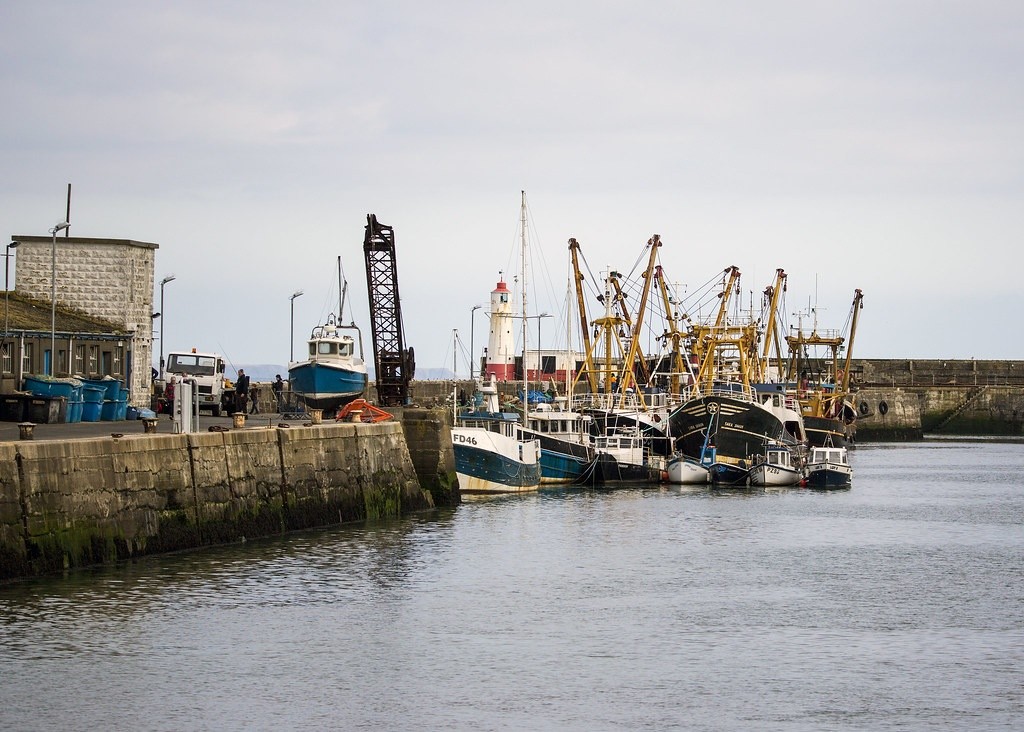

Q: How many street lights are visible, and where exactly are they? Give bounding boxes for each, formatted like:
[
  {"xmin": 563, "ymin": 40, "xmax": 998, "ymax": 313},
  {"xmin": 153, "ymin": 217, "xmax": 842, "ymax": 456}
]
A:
[
  {"xmin": 470, "ymin": 304, "xmax": 482, "ymax": 383},
  {"xmin": 49, "ymin": 221, "xmax": 72, "ymax": 377},
  {"xmin": 159, "ymin": 273, "xmax": 176, "ymax": 379},
  {"xmin": 285, "ymin": 289, "xmax": 304, "ymax": 414},
  {"xmin": 538, "ymin": 312, "xmax": 548, "ymax": 384},
  {"xmin": 5, "ymin": 240, "xmax": 22, "ymax": 334}
]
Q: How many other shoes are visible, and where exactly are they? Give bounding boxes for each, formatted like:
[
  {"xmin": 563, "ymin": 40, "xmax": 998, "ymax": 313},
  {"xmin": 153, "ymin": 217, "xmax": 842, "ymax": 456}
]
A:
[
  {"xmin": 255, "ymin": 411, "xmax": 258, "ymax": 415},
  {"xmin": 169, "ymin": 417, "xmax": 173, "ymax": 421},
  {"xmin": 249, "ymin": 412, "xmax": 252, "ymax": 414}
]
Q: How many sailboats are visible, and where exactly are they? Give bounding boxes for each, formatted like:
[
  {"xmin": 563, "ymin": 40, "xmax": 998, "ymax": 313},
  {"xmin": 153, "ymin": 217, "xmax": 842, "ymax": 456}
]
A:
[{"xmin": 449, "ymin": 187, "xmax": 867, "ymax": 498}]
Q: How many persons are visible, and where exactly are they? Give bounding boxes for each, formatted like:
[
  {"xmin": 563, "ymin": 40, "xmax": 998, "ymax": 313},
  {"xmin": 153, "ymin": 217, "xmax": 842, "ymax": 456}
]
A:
[
  {"xmin": 232, "ymin": 369, "xmax": 248, "ymax": 420},
  {"xmin": 248, "ymin": 384, "xmax": 259, "ymax": 415},
  {"xmin": 165, "ymin": 376, "xmax": 178, "ymax": 420},
  {"xmin": 272, "ymin": 375, "xmax": 283, "ymax": 413}
]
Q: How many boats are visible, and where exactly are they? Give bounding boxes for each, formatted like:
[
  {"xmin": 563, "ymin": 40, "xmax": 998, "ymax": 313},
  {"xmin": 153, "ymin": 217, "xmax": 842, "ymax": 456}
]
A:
[{"xmin": 287, "ymin": 255, "xmax": 370, "ymax": 411}]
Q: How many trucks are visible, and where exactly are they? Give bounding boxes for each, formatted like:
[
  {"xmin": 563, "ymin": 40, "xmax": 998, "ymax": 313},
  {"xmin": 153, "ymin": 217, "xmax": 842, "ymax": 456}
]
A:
[{"xmin": 164, "ymin": 348, "xmax": 250, "ymax": 416}]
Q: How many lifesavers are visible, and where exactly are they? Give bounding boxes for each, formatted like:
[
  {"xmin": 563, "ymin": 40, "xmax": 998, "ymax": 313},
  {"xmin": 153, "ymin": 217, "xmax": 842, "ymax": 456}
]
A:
[
  {"xmin": 837, "ymin": 370, "xmax": 844, "ymax": 380},
  {"xmin": 879, "ymin": 401, "xmax": 888, "ymax": 415},
  {"xmin": 860, "ymin": 402, "xmax": 869, "ymax": 414}
]
